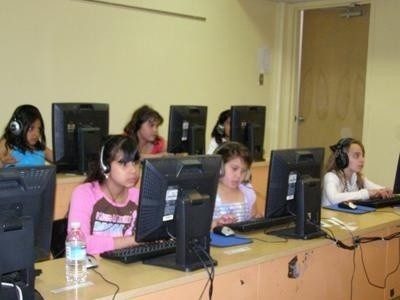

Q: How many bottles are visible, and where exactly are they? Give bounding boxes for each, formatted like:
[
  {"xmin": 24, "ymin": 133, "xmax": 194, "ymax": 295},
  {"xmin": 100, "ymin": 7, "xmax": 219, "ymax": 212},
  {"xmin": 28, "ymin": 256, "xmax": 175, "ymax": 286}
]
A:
[{"xmin": 65, "ymin": 222, "xmax": 88, "ymax": 284}]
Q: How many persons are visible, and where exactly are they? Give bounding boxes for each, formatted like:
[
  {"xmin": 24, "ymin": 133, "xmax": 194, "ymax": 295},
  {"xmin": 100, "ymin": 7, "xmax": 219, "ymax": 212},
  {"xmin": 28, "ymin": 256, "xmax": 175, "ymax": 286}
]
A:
[
  {"xmin": 320, "ymin": 137, "xmax": 396, "ymax": 206},
  {"xmin": 207, "ymin": 110, "xmax": 232, "ymax": 155},
  {"xmin": 0, "ymin": 104, "xmax": 54, "ymax": 168},
  {"xmin": 66, "ymin": 135, "xmax": 177, "ymax": 256},
  {"xmin": 123, "ymin": 105, "xmax": 171, "ymax": 160},
  {"xmin": 209, "ymin": 141, "xmax": 262, "ymax": 231}
]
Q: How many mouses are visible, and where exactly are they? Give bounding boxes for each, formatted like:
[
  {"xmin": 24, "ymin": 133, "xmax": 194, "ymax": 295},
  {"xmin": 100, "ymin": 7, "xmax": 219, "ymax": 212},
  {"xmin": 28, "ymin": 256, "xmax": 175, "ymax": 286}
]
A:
[
  {"xmin": 213, "ymin": 225, "xmax": 235, "ymax": 237},
  {"xmin": 338, "ymin": 201, "xmax": 358, "ymax": 210},
  {"xmin": 86, "ymin": 253, "xmax": 98, "ymax": 269}
]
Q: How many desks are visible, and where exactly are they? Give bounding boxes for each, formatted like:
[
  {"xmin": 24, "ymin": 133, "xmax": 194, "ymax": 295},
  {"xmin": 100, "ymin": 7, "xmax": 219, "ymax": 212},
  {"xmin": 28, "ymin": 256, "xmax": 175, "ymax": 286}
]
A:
[{"xmin": 54, "ymin": 160, "xmax": 269, "ymax": 222}]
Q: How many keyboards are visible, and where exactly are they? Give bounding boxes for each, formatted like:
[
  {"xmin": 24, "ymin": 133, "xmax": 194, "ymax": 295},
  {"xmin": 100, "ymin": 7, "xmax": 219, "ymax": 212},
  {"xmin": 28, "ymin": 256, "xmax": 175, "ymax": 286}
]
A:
[
  {"xmin": 225, "ymin": 215, "xmax": 295, "ymax": 232},
  {"xmin": 352, "ymin": 196, "xmax": 400, "ymax": 208},
  {"xmin": 99, "ymin": 240, "xmax": 176, "ymax": 263}
]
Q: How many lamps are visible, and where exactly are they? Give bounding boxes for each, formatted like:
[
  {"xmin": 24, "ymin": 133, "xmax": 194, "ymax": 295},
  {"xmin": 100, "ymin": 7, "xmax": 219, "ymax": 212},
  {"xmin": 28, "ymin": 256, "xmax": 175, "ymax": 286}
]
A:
[{"xmin": 259, "ymin": 49, "xmax": 273, "ymax": 85}]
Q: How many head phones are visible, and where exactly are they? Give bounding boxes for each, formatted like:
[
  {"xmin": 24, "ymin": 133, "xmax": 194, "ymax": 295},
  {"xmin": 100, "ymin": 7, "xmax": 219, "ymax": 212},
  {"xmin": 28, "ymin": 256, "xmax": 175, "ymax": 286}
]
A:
[
  {"xmin": 213, "ymin": 142, "xmax": 252, "ymax": 186},
  {"xmin": 9, "ymin": 105, "xmax": 23, "ymax": 136},
  {"xmin": 334, "ymin": 138, "xmax": 349, "ymax": 169},
  {"xmin": 99, "ymin": 135, "xmax": 116, "ymax": 174},
  {"xmin": 217, "ymin": 123, "xmax": 224, "ymax": 135}
]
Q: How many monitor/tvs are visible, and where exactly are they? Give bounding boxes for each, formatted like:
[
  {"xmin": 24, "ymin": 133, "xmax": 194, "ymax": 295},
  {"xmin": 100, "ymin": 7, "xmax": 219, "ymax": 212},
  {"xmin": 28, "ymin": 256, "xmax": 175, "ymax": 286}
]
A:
[
  {"xmin": 135, "ymin": 154, "xmax": 222, "ymax": 272},
  {"xmin": 230, "ymin": 105, "xmax": 266, "ymax": 161},
  {"xmin": 393, "ymin": 155, "xmax": 400, "ymax": 194},
  {"xmin": 0, "ymin": 166, "xmax": 56, "ymax": 300},
  {"xmin": 52, "ymin": 103, "xmax": 109, "ymax": 175},
  {"xmin": 167, "ymin": 105, "xmax": 207, "ymax": 155},
  {"xmin": 264, "ymin": 147, "xmax": 326, "ymax": 239}
]
